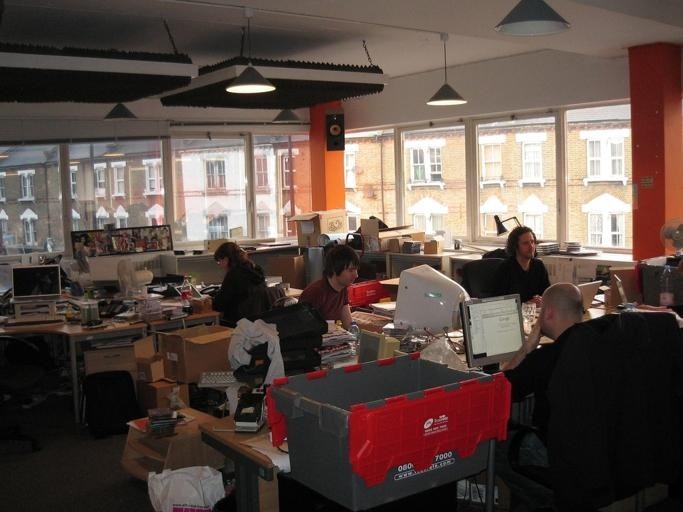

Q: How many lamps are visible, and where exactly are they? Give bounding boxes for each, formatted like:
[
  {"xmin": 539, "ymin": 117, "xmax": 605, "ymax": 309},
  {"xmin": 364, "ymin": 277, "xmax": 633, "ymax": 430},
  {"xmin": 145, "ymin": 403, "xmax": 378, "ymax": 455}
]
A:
[
  {"xmin": 427, "ymin": 32, "xmax": 469, "ymax": 106},
  {"xmin": 225, "ymin": 7, "xmax": 277, "ymax": 93},
  {"xmin": 492, "ymin": 0, "xmax": 572, "ymax": 37},
  {"xmin": 104, "ymin": 99, "xmax": 139, "ymax": 120},
  {"xmin": 494, "ymin": 214, "xmax": 522, "ymax": 236},
  {"xmin": 273, "ymin": 105, "xmax": 304, "ymax": 124}
]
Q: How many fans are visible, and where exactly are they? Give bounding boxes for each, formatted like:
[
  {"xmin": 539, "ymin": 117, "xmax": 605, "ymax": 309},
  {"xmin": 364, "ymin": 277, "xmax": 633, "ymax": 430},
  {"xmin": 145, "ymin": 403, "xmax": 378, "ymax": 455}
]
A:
[{"xmin": 659, "ymin": 216, "xmax": 683, "ymax": 256}]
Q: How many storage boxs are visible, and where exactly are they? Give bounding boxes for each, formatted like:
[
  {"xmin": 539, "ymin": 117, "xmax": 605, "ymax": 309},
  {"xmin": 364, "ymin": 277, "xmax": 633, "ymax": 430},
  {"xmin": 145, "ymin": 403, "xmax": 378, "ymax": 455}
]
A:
[
  {"xmin": 361, "ymin": 219, "xmax": 426, "ymax": 253},
  {"xmin": 149, "ymin": 323, "xmax": 235, "ymax": 385},
  {"xmin": 264, "ymin": 354, "xmax": 508, "ymax": 512},
  {"xmin": 288, "ymin": 209, "xmax": 350, "ymax": 245},
  {"xmin": 138, "ymin": 355, "xmax": 165, "ymax": 384},
  {"xmin": 641, "ymin": 252, "xmax": 683, "ymax": 309},
  {"xmin": 136, "ymin": 379, "xmax": 191, "ymax": 411}
]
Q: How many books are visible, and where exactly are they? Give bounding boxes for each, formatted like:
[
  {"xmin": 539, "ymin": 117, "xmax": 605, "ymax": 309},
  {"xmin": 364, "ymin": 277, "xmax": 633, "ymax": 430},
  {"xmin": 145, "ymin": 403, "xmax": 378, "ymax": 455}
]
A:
[
  {"xmin": 314, "ymin": 322, "xmax": 357, "ymax": 366},
  {"xmin": 382, "ymin": 322, "xmax": 418, "ymax": 352},
  {"xmin": 127, "ymin": 404, "xmax": 198, "ymax": 440},
  {"xmin": 92, "ymin": 339, "xmax": 134, "ymax": 348},
  {"xmin": 537, "ymin": 239, "xmax": 582, "ymax": 256},
  {"xmin": 60, "ymin": 260, "xmax": 188, "ymax": 328}
]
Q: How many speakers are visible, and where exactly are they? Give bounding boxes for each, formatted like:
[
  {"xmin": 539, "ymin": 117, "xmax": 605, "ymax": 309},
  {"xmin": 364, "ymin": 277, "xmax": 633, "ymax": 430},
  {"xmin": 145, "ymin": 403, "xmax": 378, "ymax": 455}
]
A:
[{"xmin": 326, "ymin": 109, "xmax": 345, "ymax": 151}]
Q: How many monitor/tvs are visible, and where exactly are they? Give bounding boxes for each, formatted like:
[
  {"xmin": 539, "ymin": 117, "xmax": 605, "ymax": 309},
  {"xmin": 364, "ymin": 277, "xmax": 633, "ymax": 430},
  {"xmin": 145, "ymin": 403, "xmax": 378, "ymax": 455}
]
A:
[
  {"xmin": 459, "ymin": 293, "xmax": 525, "ymax": 374},
  {"xmin": 393, "ymin": 264, "xmax": 470, "ymax": 336},
  {"xmin": 360, "ymin": 329, "xmax": 400, "ymax": 363},
  {"xmin": 12, "ymin": 264, "xmax": 61, "ymax": 298}
]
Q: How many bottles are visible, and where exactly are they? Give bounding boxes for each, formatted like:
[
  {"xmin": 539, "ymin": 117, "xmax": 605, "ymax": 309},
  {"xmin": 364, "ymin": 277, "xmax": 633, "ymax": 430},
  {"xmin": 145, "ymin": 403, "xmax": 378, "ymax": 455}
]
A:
[
  {"xmin": 657, "ymin": 264, "xmax": 675, "ymax": 308},
  {"xmin": 81, "ymin": 291, "xmax": 91, "ymax": 323},
  {"xmin": 180, "ymin": 275, "xmax": 193, "ymax": 315},
  {"xmin": 348, "ymin": 321, "xmax": 359, "ymax": 335}
]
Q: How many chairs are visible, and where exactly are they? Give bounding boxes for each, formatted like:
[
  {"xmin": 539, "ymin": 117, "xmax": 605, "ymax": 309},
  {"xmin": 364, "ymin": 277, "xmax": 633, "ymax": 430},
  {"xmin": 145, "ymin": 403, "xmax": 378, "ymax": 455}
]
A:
[
  {"xmin": 272, "ymin": 295, "xmax": 303, "ymax": 311},
  {"xmin": 501, "ymin": 314, "xmax": 682, "ymax": 511},
  {"xmin": 240, "ymin": 287, "xmax": 284, "ymax": 319},
  {"xmin": 460, "ymin": 257, "xmax": 505, "ymax": 294}
]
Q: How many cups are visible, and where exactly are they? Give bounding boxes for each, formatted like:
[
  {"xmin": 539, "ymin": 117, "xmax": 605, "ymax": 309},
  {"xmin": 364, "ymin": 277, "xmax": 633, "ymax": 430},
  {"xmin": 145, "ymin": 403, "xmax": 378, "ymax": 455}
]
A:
[
  {"xmin": 522, "ymin": 302, "xmax": 535, "ymax": 332},
  {"xmin": 454, "ymin": 239, "xmax": 462, "ymax": 250}
]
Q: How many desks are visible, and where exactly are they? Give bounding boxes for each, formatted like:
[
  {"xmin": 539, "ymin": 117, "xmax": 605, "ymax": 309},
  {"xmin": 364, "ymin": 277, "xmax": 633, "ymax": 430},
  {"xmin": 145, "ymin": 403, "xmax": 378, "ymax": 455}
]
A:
[
  {"xmin": 146, "ymin": 285, "xmax": 305, "ymax": 331},
  {"xmin": 158, "ymin": 245, "xmax": 301, "ymax": 281},
  {"xmin": 0, "ymin": 318, "xmax": 149, "ymax": 425},
  {"xmin": 175, "ymin": 402, "xmax": 291, "ymax": 511}
]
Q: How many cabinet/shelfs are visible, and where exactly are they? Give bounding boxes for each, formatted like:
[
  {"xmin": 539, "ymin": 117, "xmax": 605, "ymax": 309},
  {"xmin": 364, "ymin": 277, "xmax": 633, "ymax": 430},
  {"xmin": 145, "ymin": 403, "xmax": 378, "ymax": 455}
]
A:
[
  {"xmin": 118, "ymin": 408, "xmax": 228, "ymax": 486},
  {"xmin": 80, "ymin": 333, "xmax": 156, "ymax": 384},
  {"xmin": 355, "ymin": 251, "xmax": 389, "ymax": 278},
  {"xmin": 387, "ymin": 252, "xmax": 446, "ymax": 283},
  {"xmin": 448, "ymin": 256, "xmax": 482, "ymax": 285}
]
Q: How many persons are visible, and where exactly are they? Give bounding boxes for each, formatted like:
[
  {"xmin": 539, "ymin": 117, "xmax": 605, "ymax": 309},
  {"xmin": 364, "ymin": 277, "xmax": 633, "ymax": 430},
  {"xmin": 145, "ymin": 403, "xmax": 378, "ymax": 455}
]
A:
[
  {"xmin": 69, "ymin": 227, "xmax": 171, "ymax": 257},
  {"xmin": 203, "ymin": 242, "xmax": 271, "ymax": 328},
  {"xmin": 479, "ymin": 226, "xmax": 551, "ymax": 305},
  {"xmin": 297, "ymin": 244, "xmax": 359, "ymax": 329},
  {"xmin": 500, "ymin": 280, "xmax": 585, "ymax": 512}
]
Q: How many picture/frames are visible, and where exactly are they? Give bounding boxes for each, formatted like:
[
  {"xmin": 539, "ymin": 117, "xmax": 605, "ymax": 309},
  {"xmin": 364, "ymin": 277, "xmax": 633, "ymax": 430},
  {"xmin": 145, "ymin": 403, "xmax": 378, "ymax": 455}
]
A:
[{"xmin": 70, "ymin": 225, "xmax": 173, "ymax": 259}]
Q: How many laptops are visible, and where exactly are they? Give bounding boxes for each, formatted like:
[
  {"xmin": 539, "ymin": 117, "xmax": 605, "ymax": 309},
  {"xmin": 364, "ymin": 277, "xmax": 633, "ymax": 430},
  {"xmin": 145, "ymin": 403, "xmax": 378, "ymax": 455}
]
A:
[
  {"xmin": 613, "ymin": 273, "xmax": 639, "ymax": 310},
  {"xmin": 535, "ymin": 280, "xmax": 603, "ymax": 313}
]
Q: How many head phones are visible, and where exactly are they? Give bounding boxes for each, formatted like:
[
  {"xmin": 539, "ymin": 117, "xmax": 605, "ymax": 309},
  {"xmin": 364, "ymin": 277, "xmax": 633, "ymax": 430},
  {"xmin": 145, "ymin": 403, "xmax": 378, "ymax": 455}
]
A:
[{"xmin": 508, "ymin": 227, "xmax": 537, "ymax": 257}]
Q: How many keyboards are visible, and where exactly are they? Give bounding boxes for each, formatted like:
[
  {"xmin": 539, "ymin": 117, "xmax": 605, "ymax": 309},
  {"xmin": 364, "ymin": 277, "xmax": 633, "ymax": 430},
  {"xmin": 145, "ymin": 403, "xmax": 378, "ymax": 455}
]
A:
[
  {"xmin": 198, "ymin": 370, "xmax": 237, "ymax": 387},
  {"xmin": 8, "ymin": 314, "xmax": 65, "ymax": 326}
]
